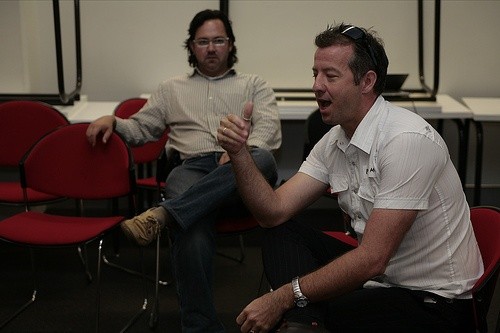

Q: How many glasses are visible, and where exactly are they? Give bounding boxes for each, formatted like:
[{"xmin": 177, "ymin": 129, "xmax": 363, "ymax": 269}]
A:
[
  {"xmin": 328, "ymin": 25, "xmax": 379, "ymax": 70},
  {"xmin": 191, "ymin": 37, "xmax": 230, "ymax": 47}
]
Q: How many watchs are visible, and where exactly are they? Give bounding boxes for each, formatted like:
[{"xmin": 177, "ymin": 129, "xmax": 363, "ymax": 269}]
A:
[{"xmin": 291, "ymin": 276, "xmax": 309, "ymax": 308}]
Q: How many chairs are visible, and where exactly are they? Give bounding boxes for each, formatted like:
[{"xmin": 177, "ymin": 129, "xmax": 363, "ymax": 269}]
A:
[{"xmin": 0, "ymin": 97, "xmax": 500, "ymax": 333}]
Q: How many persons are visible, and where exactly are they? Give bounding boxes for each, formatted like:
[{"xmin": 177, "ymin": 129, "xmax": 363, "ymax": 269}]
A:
[
  {"xmin": 86, "ymin": 9, "xmax": 283, "ymax": 333},
  {"xmin": 218, "ymin": 21, "xmax": 485, "ymax": 333}
]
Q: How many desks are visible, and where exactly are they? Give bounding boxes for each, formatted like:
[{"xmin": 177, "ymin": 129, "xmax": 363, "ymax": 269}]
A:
[{"xmin": 0, "ymin": 0, "xmax": 500, "ymax": 207}]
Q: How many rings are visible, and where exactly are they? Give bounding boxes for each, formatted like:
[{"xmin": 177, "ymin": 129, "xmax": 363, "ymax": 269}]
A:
[
  {"xmin": 243, "ymin": 115, "xmax": 253, "ymax": 122},
  {"xmin": 222, "ymin": 126, "xmax": 228, "ymax": 134}
]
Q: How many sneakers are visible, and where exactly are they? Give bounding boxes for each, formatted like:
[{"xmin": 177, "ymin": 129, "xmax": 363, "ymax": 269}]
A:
[{"xmin": 120, "ymin": 208, "xmax": 164, "ymax": 245}]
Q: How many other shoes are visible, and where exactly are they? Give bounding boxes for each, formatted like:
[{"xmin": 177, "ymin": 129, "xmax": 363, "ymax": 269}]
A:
[{"xmin": 270, "ymin": 320, "xmax": 329, "ymax": 333}]
